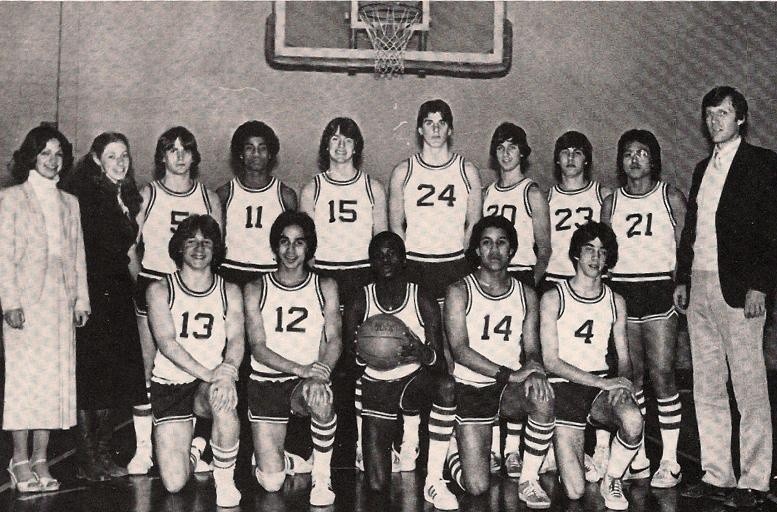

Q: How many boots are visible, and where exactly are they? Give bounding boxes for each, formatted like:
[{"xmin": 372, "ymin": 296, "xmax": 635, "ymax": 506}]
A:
[
  {"xmin": 95, "ymin": 406, "xmax": 129, "ymax": 480},
  {"xmin": 72, "ymin": 405, "xmax": 112, "ymax": 484}
]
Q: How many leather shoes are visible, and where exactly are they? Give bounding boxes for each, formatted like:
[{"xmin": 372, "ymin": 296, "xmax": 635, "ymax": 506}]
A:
[
  {"xmin": 678, "ymin": 480, "xmax": 719, "ymax": 499},
  {"xmin": 721, "ymin": 487, "xmax": 768, "ymax": 512}
]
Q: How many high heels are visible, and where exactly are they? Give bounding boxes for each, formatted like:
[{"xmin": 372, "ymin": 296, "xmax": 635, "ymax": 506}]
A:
[
  {"xmin": 27, "ymin": 457, "xmax": 59, "ymax": 494},
  {"xmin": 5, "ymin": 456, "xmax": 39, "ymax": 494}
]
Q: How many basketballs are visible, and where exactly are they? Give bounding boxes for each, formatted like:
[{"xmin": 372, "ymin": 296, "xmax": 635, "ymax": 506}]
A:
[{"xmin": 356, "ymin": 315, "xmax": 407, "ymax": 371}]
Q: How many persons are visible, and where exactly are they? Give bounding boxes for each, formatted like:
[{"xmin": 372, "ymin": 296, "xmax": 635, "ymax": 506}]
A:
[
  {"xmin": 478, "ymin": 121, "xmax": 553, "ymax": 480},
  {"xmin": 211, "ymin": 121, "xmax": 299, "ymax": 476},
  {"xmin": 536, "ymin": 131, "xmax": 611, "ymax": 485},
  {"xmin": 597, "ymin": 128, "xmax": 694, "ymax": 489},
  {"xmin": 57, "ymin": 130, "xmax": 145, "ymax": 487},
  {"xmin": 668, "ymin": 84, "xmax": 776, "ymax": 508},
  {"xmin": 242, "ymin": 208, "xmax": 343, "ymax": 507},
  {"xmin": 0, "ymin": 125, "xmax": 91, "ymax": 494},
  {"xmin": 443, "ymin": 213, "xmax": 555, "ymax": 511},
  {"xmin": 386, "ymin": 100, "xmax": 482, "ymax": 472},
  {"xmin": 539, "ymin": 221, "xmax": 644, "ymax": 511},
  {"xmin": 126, "ymin": 125, "xmax": 220, "ymax": 479},
  {"xmin": 293, "ymin": 117, "xmax": 386, "ymax": 473},
  {"xmin": 341, "ymin": 231, "xmax": 461, "ymax": 511},
  {"xmin": 141, "ymin": 213, "xmax": 243, "ymax": 510}
]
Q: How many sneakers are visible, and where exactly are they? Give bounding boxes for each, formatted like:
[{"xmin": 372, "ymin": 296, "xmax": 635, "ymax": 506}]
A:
[
  {"xmin": 127, "ymin": 441, "xmax": 153, "ymax": 475},
  {"xmin": 307, "ymin": 466, "xmax": 337, "ymax": 506},
  {"xmin": 597, "ymin": 473, "xmax": 628, "ymax": 510},
  {"xmin": 422, "ymin": 476, "xmax": 460, "ymax": 510},
  {"xmin": 648, "ymin": 459, "xmax": 684, "ymax": 491},
  {"xmin": 490, "ymin": 452, "xmax": 501, "ymax": 474},
  {"xmin": 504, "ymin": 449, "xmax": 523, "ymax": 479},
  {"xmin": 582, "ymin": 452, "xmax": 600, "ymax": 482},
  {"xmin": 355, "ymin": 440, "xmax": 420, "ymax": 474},
  {"xmin": 516, "ymin": 476, "xmax": 552, "ymax": 510},
  {"xmin": 192, "ymin": 435, "xmax": 207, "ymax": 453},
  {"xmin": 281, "ymin": 449, "xmax": 312, "ymax": 476},
  {"xmin": 593, "ymin": 446, "xmax": 610, "ymax": 479},
  {"xmin": 211, "ymin": 465, "xmax": 241, "ymax": 509},
  {"xmin": 620, "ymin": 458, "xmax": 651, "ymax": 481}
]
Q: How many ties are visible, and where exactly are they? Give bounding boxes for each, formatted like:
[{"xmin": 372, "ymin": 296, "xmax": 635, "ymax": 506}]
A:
[{"xmin": 713, "ymin": 151, "xmax": 725, "ymax": 172}]
